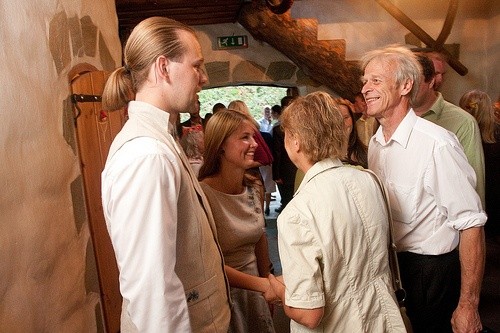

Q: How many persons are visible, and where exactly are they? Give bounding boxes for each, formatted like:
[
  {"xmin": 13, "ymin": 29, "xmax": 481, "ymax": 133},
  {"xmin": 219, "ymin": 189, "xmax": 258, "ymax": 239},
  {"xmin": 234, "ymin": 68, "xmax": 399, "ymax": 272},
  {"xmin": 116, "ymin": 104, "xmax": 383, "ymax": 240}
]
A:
[
  {"xmin": 102, "ymin": 17, "xmax": 242, "ymax": 333},
  {"xmin": 460, "ymin": 91, "xmax": 500, "ymax": 333},
  {"xmin": 334, "ymin": 92, "xmax": 378, "ymax": 168},
  {"xmin": 198, "ymin": 110, "xmax": 285, "ymax": 333},
  {"xmin": 177, "ymin": 100, "xmax": 274, "ymax": 178},
  {"xmin": 263, "ymin": 91, "xmax": 409, "ymax": 333},
  {"xmin": 258, "ymin": 84, "xmax": 300, "ymax": 216},
  {"xmin": 362, "ymin": 45, "xmax": 487, "ymax": 333},
  {"xmin": 410, "ymin": 53, "xmax": 486, "ymax": 210}
]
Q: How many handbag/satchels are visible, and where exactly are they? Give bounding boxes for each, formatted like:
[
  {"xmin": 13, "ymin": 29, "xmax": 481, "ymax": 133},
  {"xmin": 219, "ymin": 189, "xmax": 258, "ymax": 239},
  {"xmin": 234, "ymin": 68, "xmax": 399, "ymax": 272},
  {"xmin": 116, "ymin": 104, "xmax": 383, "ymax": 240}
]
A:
[{"xmin": 400, "ymin": 307, "xmax": 415, "ymax": 333}]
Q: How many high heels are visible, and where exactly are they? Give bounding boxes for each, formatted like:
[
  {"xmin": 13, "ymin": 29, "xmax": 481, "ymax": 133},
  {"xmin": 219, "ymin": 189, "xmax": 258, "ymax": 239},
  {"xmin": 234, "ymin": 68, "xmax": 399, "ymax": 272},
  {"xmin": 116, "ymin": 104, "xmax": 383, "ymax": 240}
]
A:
[{"xmin": 265, "ymin": 207, "xmax": 270, "ymax": 215}]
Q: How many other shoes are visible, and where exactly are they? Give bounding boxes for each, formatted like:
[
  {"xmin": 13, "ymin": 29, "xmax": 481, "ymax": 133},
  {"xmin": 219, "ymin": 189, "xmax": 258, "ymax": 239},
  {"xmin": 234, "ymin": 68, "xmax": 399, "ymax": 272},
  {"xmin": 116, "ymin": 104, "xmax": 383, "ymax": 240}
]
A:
[
  {"xmin": 275, "ymin": 207, "xmax": 283, "ymax": 213},
  {"xmin": 270, "ymin": 195, "xmax": 276, "ymax": 198},
  {"xmin": 270, "ymin": 199, "xmax": 276, "ymax": 201}
]
